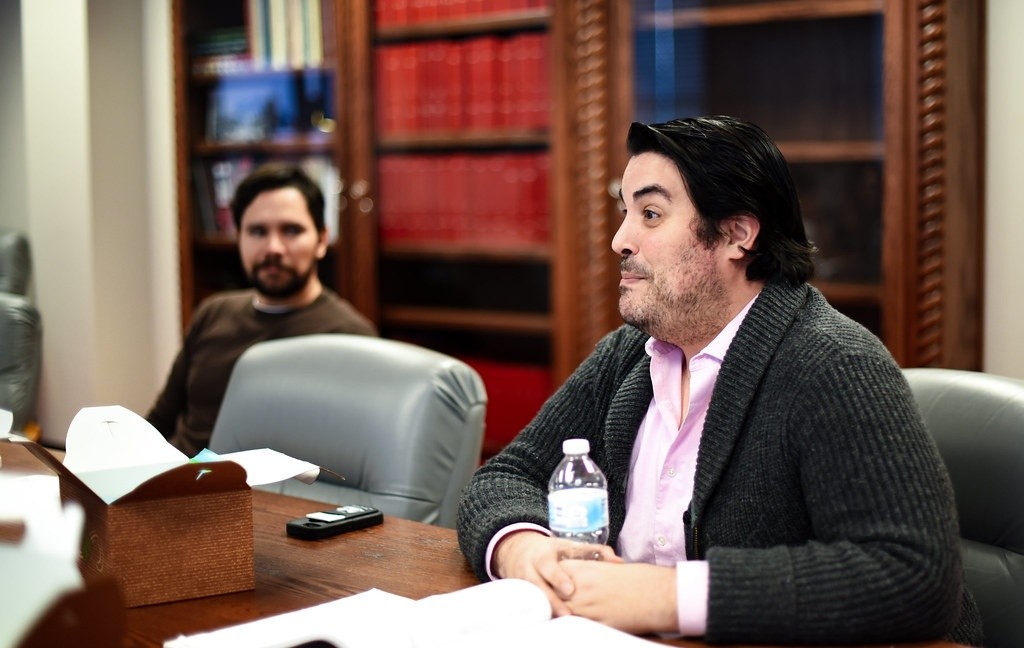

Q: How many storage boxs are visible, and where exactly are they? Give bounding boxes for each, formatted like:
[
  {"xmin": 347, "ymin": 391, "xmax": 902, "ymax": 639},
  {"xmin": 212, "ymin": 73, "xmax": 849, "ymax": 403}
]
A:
[{"xmin": 17, "ymin": 403, "xmax": 345, "ymax": 607}]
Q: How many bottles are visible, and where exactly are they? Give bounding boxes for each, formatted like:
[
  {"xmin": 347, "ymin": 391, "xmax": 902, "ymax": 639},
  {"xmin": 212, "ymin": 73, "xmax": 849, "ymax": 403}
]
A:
[{"xmin": 548, "ymin": 439, "xmax": 609, "ymax": 560}]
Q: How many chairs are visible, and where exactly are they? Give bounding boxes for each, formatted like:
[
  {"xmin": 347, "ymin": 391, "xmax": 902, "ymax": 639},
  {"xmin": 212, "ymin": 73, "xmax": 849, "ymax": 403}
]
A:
[
  {"xmin": 0, "ymin": 232, "xmax": 46, "ymax": 435},
  {"xmin": 212, "ymin": 333, "xmax": 486, "ymax": 527},
  {"xmin": 899, "ymin": 367, "xmax": 1024, "ymax": 648}
]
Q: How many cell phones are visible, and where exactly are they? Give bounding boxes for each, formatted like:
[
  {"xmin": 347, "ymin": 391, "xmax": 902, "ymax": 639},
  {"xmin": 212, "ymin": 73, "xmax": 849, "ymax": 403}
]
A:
[{"xmin": 285, "ymin": 504, "xmax": 384, "ymax": 540}]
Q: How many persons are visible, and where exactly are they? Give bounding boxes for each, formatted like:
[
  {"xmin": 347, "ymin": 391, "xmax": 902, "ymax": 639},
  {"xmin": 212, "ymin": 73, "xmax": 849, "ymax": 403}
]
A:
[
  {"xmin": 457, "ymin": 115, "xmax": 984, "ymax": 644},
  {"xmin": 144, "ymin": 162, "xmax": 377, "ymax": 459}
]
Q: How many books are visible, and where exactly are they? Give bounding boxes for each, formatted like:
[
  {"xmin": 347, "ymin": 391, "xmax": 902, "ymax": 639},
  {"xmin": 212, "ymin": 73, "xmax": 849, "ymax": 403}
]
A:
[
  {"xmin": 164, "ymin": 579, "xmax": 674, "ymax": 648},
  {"xmin": 204, "ymin": 0, "xmax": 338, "ymax": 234}
]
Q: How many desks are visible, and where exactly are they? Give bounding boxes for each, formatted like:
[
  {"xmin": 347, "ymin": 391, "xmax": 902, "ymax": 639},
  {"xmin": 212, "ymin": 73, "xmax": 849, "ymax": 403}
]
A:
[{"xmin": 0, "ymin": 440, "xmax": 983, "ymax": 648}]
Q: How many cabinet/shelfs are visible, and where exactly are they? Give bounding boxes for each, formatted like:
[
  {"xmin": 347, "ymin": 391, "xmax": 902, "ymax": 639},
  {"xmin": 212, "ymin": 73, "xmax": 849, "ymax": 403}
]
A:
[
  {"xmin": 584, "ymin": 0, "xmax": 985, "ymax": 420},
  {"xmin": 173, "ymin": 1, "xmax": 353, "ymax": 346},
  {"xmin": 346, "ymin": 1, "xmax": 583, "ymax": 456}
]
